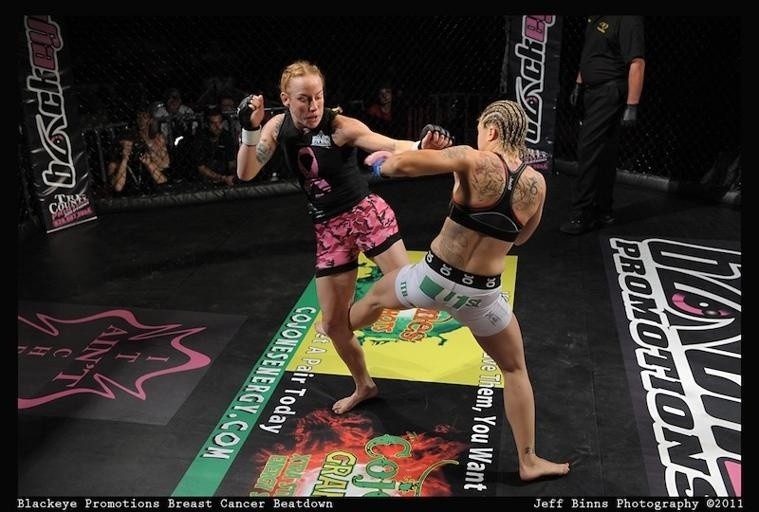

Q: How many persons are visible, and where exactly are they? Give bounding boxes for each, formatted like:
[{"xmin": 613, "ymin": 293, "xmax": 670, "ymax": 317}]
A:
[
  {"xmin": 560, "ymin": 16, "xmax": 646, "ymax": 235},
  {"xmin": 234, "ymin": 62, "xmax": 457, "ymax": 416},
  {"xmin": 314, "ymin": 100, "xmax": 570, "ymax": 482},
  {"xmin": 105, "ymin": 89, "xmax": 390, "ymax": 196}
]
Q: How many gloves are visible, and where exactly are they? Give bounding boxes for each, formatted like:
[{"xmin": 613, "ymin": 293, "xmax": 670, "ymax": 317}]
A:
[
  {"xmin": 621, "ymin": 104, "xmax": 637, "ymax": 127},
  {"xmin": 570, "ymin": 83, "xmax": 581, "ymax": 106}
]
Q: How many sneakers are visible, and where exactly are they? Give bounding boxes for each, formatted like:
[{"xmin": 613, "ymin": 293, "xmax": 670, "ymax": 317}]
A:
[
  {"xmin": 600, "ymin": 215, "xmax": 615, "ymax": 225},
  {"xmin": 560, "ymin": 220, "xmax": 594, "ymax": 233}
]
